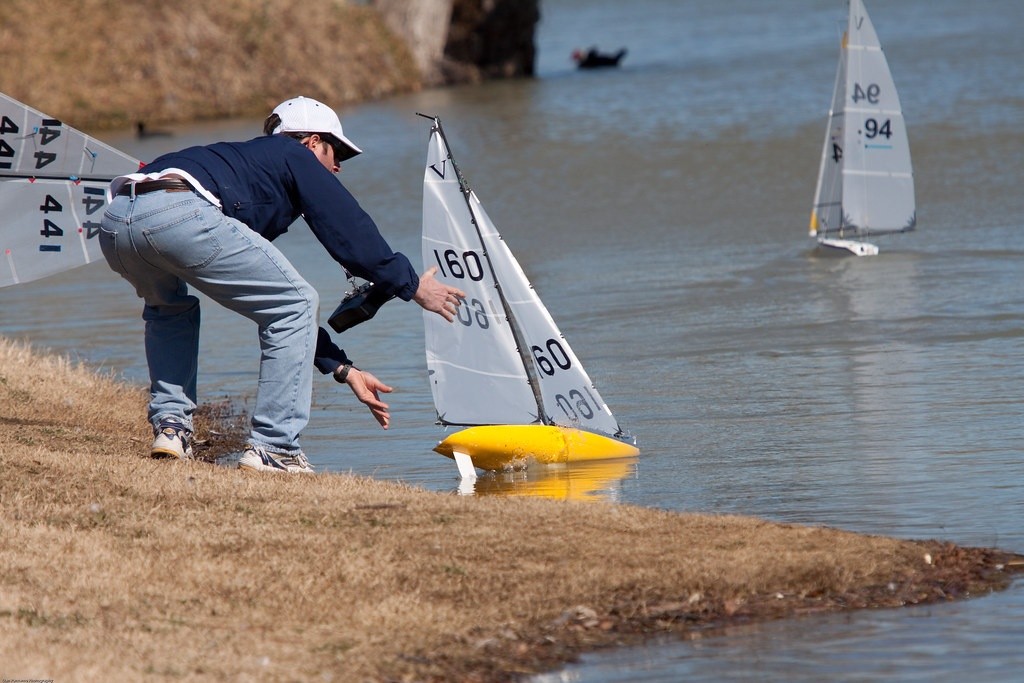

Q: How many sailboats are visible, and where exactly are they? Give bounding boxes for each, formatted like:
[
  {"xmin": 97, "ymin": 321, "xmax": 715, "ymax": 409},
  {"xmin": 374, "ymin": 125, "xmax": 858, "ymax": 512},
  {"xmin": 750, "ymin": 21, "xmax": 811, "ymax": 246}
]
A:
[
  {"xmin": 806, "ymin": 1, "xmax": 920, "ymax": 262},
  {"xmin": 413, "ymin": 111, "xmax": 644, "ymax": 480}
]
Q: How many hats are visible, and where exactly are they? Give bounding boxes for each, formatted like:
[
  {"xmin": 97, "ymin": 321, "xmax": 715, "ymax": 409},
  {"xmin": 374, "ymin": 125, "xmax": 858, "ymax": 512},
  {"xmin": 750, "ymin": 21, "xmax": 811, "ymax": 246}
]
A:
[{"xmin": 267, "ymin": 96, "xmax": 362, "ymax": 162}]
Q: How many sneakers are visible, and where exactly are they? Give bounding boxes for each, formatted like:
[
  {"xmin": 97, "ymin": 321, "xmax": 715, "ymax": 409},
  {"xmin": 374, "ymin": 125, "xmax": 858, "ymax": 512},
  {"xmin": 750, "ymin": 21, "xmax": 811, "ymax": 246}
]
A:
[
  {"xmin": 238, "ymin": 443, "xmax": 315, "ymax": 474},
  {"xmin": 150, "ymin": 429, "xmax": 194, "ymax": 462}
]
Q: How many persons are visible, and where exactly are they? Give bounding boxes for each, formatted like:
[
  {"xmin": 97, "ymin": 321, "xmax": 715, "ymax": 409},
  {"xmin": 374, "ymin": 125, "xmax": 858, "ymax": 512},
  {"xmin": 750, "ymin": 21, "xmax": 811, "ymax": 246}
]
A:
[{"xmin": 99, "ymin": 96, "xmax": 465, "ymax": 475}]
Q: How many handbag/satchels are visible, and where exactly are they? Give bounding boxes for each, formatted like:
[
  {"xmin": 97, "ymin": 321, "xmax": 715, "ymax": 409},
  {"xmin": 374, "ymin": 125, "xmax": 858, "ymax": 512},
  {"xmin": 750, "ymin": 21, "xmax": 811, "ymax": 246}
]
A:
[{"xmin": 328, "ymin": 281, "xmax": 394, "ymax": 334}]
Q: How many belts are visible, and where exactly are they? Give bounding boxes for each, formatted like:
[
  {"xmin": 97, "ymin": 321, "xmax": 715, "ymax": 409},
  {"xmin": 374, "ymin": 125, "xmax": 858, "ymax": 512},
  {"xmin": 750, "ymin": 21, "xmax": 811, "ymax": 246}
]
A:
[{"xmin": 115, "ymin": 179, "xmax": 195, "ymax": 196}]
